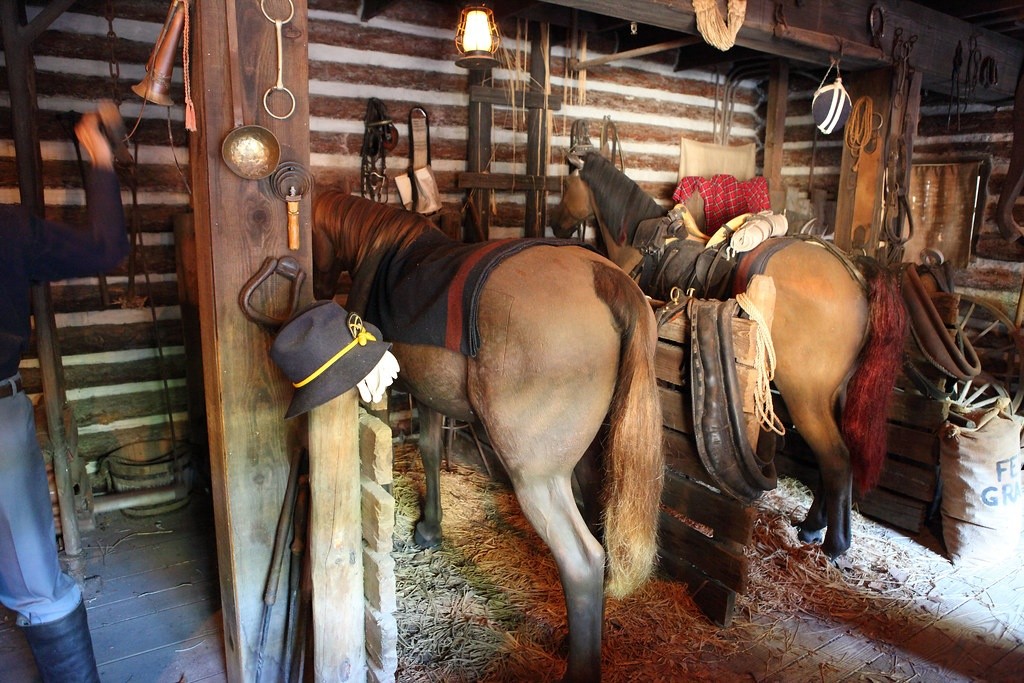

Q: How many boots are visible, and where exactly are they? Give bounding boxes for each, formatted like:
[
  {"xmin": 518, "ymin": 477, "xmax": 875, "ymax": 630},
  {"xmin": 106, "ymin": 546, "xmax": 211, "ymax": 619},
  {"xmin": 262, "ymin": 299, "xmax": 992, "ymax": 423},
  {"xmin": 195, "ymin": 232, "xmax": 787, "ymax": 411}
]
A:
[{"xmin": 15, "ymin": 592, "xmax": 101, "ymax": 683}]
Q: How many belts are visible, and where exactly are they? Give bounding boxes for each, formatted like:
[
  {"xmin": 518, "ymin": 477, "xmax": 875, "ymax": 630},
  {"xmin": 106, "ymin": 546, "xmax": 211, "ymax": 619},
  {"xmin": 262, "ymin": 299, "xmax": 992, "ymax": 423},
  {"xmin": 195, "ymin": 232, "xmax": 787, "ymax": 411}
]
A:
[{"xmin": 0, "ymin": 378, "xmax": 23, "ymax": 399}]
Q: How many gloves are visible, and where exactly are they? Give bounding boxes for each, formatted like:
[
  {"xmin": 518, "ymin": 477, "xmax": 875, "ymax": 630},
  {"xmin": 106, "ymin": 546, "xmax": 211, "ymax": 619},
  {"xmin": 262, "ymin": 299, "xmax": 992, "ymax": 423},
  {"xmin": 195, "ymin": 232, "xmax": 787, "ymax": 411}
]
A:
[{"xmin": 357, "ymin": 350, "xmax": 400, "ymax": 403}]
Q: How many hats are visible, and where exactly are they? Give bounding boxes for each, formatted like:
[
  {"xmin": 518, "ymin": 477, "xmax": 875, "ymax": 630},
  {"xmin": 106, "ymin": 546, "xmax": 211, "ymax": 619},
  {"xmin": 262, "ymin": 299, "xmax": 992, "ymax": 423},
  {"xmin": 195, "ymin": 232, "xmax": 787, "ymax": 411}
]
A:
[{"xmin": 269, "ymin": 300, "xmax": 393, "ymax": 419}]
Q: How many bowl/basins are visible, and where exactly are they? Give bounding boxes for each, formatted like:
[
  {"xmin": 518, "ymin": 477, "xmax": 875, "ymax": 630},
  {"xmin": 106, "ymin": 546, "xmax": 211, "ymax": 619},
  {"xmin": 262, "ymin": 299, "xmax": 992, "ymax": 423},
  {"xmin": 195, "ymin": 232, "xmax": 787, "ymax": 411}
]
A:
[{"xmin": 222, "ymin": 124, "xmax": 279, "ymax": 180}]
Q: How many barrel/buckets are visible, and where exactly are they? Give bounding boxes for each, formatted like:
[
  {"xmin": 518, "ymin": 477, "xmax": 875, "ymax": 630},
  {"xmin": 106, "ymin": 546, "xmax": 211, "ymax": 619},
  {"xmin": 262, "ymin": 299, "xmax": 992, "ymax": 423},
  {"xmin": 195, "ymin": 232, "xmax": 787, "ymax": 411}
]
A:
[{"xmin": 107, "ymin": 439, "xmax": 190, "ymax": 517}]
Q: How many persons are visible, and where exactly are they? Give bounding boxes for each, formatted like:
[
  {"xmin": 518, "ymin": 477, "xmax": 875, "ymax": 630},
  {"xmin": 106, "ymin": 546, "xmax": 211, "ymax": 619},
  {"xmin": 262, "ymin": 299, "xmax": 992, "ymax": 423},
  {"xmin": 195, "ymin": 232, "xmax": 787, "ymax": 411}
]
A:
[{"xmin": 0, "ymin": 111, "xmax": 128, "ymax": 683}]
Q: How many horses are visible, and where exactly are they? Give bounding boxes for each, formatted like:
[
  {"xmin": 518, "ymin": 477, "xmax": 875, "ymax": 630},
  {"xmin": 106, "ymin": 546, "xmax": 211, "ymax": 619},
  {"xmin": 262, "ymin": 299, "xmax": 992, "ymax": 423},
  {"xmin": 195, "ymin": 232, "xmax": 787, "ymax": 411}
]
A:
[
  {"xmin": 550, "ymin": 143, "xmax": 911, "ymax": 565},
  {"xmin": 312, "ymin": 178, "xmax": 666, "ymax": 682}
]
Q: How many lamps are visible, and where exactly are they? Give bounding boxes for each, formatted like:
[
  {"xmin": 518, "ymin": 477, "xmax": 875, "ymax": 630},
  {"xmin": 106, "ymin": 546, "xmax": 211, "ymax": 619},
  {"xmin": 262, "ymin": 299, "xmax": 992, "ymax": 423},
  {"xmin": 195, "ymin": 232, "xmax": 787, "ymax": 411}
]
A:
[{"xmin": 454, "ymin": 0, "xmax": 502, "ymax": 69}]
